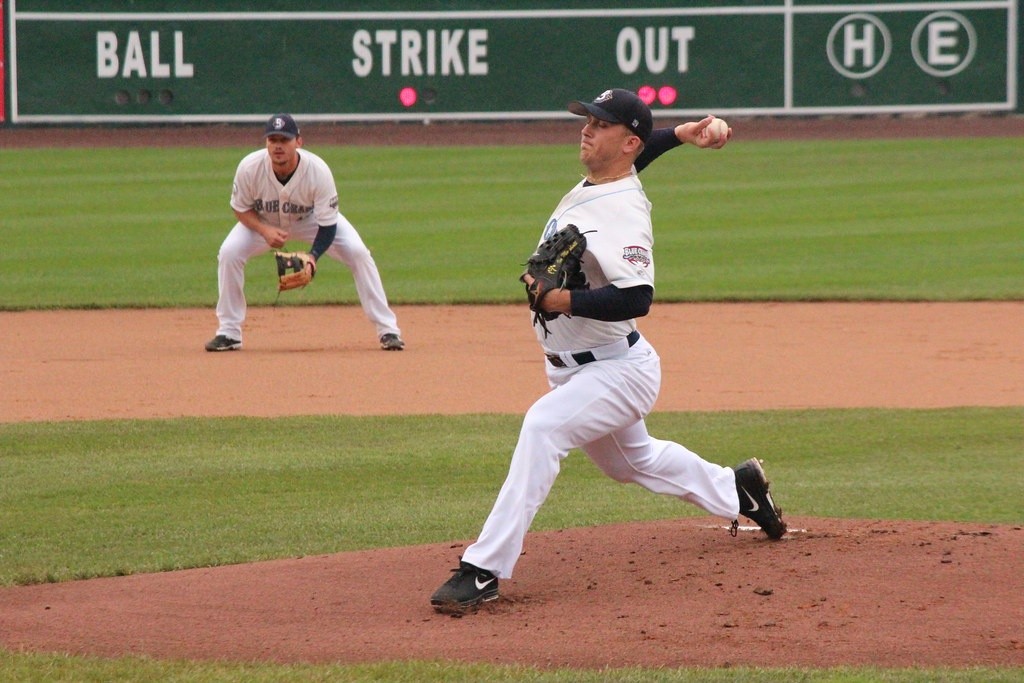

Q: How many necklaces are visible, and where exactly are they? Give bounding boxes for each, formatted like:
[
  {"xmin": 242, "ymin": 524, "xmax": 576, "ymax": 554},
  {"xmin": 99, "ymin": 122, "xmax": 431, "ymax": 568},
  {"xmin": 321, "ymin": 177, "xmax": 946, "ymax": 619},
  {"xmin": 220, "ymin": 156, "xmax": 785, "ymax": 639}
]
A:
[{"xmin": 580, "ymin": 170, "xmax": 632, "ymax": 181}]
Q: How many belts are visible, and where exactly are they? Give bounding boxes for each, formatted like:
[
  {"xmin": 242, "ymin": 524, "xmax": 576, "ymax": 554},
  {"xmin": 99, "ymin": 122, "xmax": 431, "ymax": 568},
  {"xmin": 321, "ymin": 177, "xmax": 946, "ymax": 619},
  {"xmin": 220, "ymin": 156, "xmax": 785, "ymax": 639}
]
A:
[{"xmin": 544, "ymin": 330, "xmax": 640, "ymax": 367}]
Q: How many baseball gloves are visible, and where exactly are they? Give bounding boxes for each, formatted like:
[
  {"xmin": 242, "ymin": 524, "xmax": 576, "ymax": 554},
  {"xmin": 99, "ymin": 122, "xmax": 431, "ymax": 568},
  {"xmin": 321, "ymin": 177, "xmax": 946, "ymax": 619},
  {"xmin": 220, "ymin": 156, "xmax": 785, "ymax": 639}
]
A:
[
  {"xmin": 518, "ymin": 225, "xmax": 589, "ymax": 322},
  {"xmin": 274, "ymin": 251, "xmax": 316, "ymax": 291}
]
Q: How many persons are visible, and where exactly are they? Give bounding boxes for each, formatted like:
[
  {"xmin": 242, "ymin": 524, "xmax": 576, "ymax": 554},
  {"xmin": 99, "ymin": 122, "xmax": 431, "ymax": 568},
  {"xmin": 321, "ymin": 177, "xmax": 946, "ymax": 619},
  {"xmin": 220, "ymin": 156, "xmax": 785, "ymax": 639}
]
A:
[
  {"xmin": 204, "ymin": 113, "xmax": 405, "ymax": 350},
  {"xmin": 430, "ymin": 88, "xmax": 787, "ymax": 612}
]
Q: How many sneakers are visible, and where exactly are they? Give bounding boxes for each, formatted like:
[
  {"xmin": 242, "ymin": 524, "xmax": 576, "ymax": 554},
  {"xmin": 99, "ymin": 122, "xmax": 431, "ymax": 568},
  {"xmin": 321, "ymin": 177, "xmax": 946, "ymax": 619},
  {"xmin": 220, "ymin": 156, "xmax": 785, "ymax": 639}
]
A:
[
  {"xmin": 205, "ymin": 335, "xmax": 242, "ymax": 350},
  {"xmin": 380, "ymin": 333, "xmax": 405, "ymax": 350},
  {"xmin": 430, "ymin": 555, "xmax": 499, "ymax": 614},
  {"xmin": 731, "ymin": 457, "xmax": 788, "ymax": 539}
]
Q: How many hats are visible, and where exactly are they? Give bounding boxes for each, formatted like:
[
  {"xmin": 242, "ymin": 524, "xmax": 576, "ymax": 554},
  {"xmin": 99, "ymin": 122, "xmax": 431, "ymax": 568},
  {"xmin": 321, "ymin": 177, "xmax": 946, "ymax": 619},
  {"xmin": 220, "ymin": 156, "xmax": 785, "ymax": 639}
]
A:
[
  {"xmin": 263, "ymin": 113, "xmax": 300, "ymax": 139},
  {"xmin": 568, "ymin": 88, "xmax": 653, "ymax": 144}
]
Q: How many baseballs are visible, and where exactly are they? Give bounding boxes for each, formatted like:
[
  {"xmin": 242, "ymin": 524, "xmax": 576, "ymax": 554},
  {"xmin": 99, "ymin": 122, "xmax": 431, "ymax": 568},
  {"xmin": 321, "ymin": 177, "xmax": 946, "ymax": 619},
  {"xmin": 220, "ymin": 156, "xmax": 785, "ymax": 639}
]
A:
[{"xmin": 705, "ymin": 119, "xmax": 728, "ymax": 143}]
